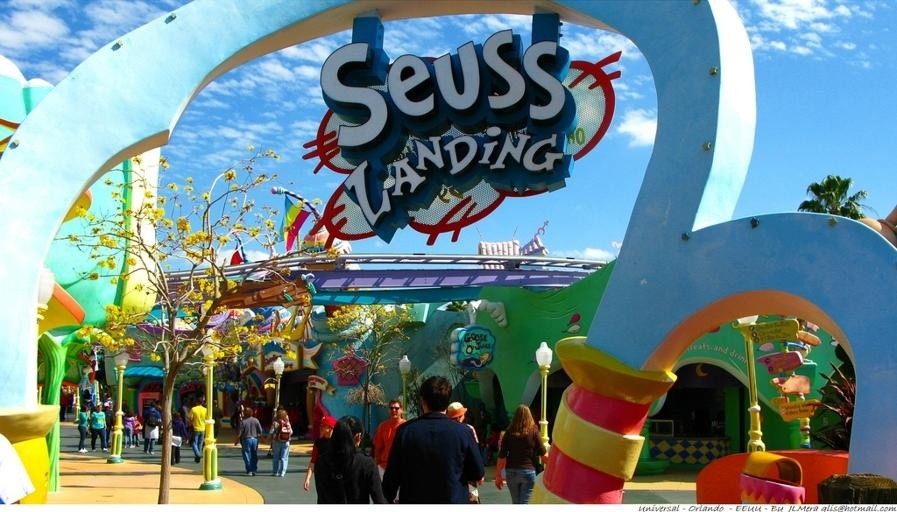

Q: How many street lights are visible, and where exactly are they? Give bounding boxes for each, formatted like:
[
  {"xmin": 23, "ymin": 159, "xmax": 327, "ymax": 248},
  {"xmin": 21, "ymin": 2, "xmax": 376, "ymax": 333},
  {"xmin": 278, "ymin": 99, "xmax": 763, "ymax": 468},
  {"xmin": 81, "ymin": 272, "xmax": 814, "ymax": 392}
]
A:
[
  {"xmin": 106, "ymin": 347, "xmax": 129, "ymax": 464},
  {"xmin": 738, "ymin": 315, "xmax": 768, "ymax": 453},
  {"xmin": 534, "ymin": 340, "xmax": 553, "ymax": 458},
  {"xmin": 200, "ymin": 328, "xmax": 224, "ymax": 490},
  {"xmin": 398, "ymin": 352, "xmax": 412, "ymax": 420},
  {"xmin": 271, "ymin": 357, "xmax": 285, "ymax": 417}
]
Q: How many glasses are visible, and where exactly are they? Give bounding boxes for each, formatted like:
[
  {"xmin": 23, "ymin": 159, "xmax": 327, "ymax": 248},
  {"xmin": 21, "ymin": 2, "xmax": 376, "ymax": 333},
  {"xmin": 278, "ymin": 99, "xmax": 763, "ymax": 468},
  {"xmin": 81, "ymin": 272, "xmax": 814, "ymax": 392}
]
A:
[{"xmin": 390, "ymin": 407, "xmax": 399, "ymax": 410}]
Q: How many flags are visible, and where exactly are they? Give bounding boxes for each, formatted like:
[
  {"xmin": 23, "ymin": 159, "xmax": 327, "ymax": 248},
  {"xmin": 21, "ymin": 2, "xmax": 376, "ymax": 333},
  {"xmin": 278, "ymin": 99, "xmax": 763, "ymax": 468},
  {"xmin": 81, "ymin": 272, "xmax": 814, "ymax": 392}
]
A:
[{"xmin": 269, "ymin": 196, "xmax": 311, "ymax": 252}]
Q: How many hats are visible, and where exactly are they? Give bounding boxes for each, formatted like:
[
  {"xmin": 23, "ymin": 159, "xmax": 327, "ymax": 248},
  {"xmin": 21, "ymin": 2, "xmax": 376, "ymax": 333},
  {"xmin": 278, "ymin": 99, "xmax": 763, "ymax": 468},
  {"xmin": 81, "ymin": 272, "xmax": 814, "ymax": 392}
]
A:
[
  {"xmin": 445, "ymin": 401, "xmax": 468, "ymax": 419},
  {"xmin": 321, "ymin": 416, "xmax": 336, "ymax": 427}
]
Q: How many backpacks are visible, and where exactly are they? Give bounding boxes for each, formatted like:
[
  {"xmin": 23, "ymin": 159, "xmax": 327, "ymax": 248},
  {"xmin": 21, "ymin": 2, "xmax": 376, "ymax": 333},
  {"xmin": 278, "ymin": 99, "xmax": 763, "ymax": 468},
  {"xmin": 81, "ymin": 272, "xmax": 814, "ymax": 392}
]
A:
[
  {"xmin": 147, "ymin": 410, "xmax": 157, "ymax": 427},
  {"xmin": 274, "ymin": 419, "xmax": 289, "ymax": 443}
]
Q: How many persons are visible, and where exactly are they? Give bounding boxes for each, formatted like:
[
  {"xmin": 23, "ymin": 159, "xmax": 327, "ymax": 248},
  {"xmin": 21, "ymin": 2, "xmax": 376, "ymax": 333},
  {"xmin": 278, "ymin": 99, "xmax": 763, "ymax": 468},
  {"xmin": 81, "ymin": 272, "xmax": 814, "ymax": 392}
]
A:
[
  {"xmin": 0, "ymin": 432, "xmax": 36, "ymax": 504},
  {"xmin": 495, "ymin": 405, "xmax": 549, "ymax": 503},
  {"xmin": 59, "ymin": 377, "xmax": 485, "ymax": 503}
]
